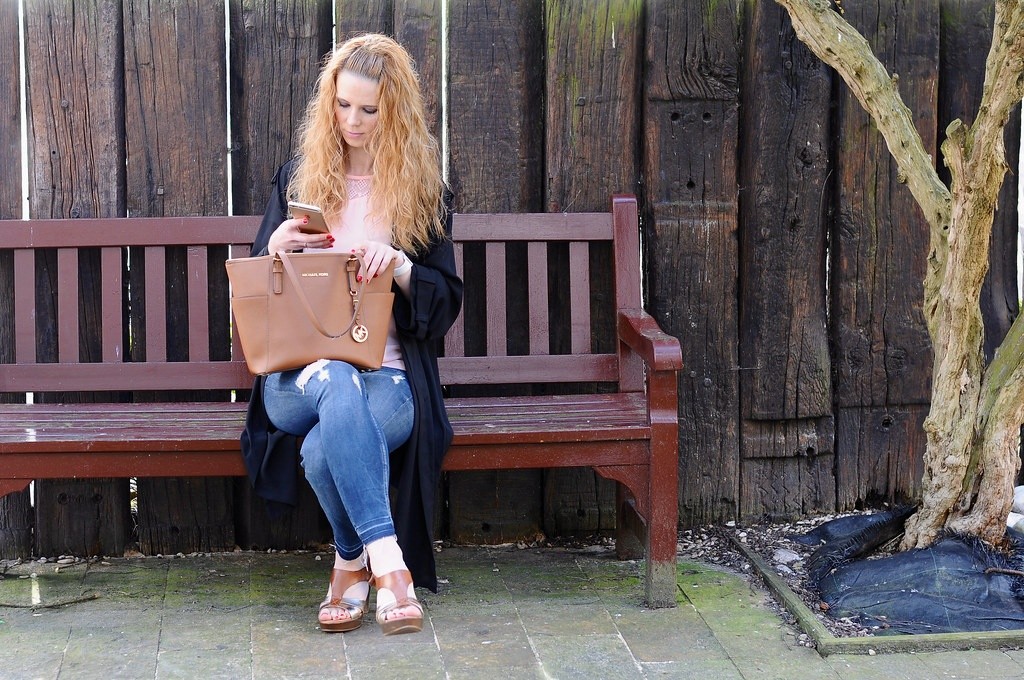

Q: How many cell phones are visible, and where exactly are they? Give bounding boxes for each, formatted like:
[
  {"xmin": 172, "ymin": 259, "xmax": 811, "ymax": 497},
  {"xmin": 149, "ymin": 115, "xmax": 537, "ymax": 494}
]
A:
[{"xmin": 288, "ymin": 201, "xmax": 330, "ymax": 234}]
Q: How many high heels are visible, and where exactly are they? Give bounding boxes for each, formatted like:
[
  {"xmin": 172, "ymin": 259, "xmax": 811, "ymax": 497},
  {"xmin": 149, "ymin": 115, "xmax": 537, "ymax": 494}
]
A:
[
  {"xmin": 368, "ymin": 570, "xmax": 424, "ymax": 636},
  {"xmin": 317, "ymin": 565, "xmax": 372, "ymax": 631}
]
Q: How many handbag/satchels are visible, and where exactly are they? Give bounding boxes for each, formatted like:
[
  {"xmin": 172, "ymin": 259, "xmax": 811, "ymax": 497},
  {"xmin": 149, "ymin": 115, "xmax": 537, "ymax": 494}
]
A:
[{"xmin": 225, "ymin": 250, "xmax": 396, "ymax": 376}]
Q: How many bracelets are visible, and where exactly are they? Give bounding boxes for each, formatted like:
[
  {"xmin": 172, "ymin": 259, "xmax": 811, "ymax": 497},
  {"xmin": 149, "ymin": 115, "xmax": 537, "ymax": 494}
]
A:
[{"xmin": 393, "ymin": 250, "xmax": 414, "ymax": 277}]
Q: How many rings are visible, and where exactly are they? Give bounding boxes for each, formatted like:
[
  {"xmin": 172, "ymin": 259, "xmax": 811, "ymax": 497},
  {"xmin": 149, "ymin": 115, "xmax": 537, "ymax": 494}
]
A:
[{"xmin": 304, "ymin": 243, "xmax": 308, "ymax": 247}]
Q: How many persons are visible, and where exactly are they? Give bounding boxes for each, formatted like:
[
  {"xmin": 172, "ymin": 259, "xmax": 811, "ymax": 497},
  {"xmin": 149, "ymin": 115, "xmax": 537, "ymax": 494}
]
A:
[{"xmin": 240, "ymin": 29, "xmax": 465, "ymax": 635}]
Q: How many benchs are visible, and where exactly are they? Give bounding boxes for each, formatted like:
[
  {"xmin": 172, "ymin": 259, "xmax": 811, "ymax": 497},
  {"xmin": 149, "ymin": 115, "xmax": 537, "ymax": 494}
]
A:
[{"xmin": 1, "ymin": 194, "xmax": 684, "ymax": 610}]
